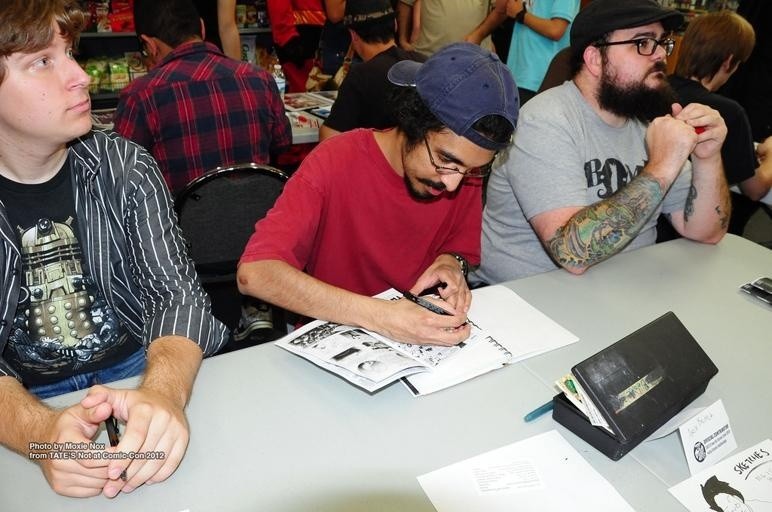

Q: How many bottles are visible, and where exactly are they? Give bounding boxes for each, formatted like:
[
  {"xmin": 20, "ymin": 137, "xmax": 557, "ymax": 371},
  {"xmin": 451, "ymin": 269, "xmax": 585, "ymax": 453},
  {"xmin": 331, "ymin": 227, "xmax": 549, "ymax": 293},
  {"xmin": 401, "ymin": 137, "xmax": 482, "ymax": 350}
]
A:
[{"xmin": 271, "ymin": 65, "xmax": 285, "ymax": 104}]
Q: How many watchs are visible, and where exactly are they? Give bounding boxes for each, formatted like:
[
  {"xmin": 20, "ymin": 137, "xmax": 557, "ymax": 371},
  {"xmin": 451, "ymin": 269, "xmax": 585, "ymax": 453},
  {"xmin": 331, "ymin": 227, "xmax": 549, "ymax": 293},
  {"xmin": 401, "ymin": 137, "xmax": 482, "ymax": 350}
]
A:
[{"xmin": 450, "ymin": 253, "xmax": 470, "ymax": 276}]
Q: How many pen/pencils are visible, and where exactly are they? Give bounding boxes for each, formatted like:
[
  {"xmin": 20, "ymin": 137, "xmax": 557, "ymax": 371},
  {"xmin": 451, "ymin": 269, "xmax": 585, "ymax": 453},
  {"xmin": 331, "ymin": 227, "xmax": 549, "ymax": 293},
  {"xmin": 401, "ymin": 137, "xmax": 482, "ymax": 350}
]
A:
[
  {"xmin": 524, "ymin": 401, "xmax": 552, "ymax": 421},
  {"xmin": 402, "ymin": 292, "xmax": 467, "ymax": 330},
  {"xmin": 91, "ymin": 379, "xmax": 129, "ymax": 483}
]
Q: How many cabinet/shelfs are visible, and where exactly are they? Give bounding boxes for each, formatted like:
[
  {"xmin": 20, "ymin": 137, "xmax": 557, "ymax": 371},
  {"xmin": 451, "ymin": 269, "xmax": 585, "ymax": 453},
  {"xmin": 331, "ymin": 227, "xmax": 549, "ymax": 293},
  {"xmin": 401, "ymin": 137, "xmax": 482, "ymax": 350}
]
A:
[
  {"xmin": 234, "ymin": 0, "xmax": 291, "ymax": 82},
  {"xmin": 69, "ymin": 0, "xmax": 156, "ymax": 109}
]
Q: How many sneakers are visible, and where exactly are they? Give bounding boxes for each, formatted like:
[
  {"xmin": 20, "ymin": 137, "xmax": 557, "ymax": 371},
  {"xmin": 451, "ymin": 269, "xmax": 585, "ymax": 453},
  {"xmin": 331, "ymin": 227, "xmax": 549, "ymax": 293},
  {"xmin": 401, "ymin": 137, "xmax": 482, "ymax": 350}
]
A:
[{"xmin": 233, "ymin": 306, "xmax": 274, "ymax": 342}]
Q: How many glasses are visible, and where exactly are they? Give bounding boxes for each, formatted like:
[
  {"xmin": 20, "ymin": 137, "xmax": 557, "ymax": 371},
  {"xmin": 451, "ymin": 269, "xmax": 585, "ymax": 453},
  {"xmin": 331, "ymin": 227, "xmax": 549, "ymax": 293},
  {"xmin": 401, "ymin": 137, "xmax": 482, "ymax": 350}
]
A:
[
  {"xmin": 594, "ymin": 36, "xmax": 675, "ymax": 58},
  {"xmin": 422, "ymin": 132, "xmax": 496, "ymax": 179},
  {"xmin": 137, "ymin": 40, "xmax": 150, "ymax": 57}
]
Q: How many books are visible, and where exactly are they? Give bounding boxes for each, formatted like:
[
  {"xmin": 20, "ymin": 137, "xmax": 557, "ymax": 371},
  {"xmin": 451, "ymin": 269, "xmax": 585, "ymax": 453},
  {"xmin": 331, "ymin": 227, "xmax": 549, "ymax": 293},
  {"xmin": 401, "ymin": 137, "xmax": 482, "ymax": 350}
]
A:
[
  {"xmin": 271, "ymin": 283, "xmax": 475, "ymax": 399},
  {"xmin": 311, "ymin": 105, "xmax": 332, "ymax": 119},
  {"xmin": 401, "ymin": 281, "xmax": 581, "ymax": 398},
  {"xmin": 283, "ymin": 90, "xmax": 338, "ymax": 112}
]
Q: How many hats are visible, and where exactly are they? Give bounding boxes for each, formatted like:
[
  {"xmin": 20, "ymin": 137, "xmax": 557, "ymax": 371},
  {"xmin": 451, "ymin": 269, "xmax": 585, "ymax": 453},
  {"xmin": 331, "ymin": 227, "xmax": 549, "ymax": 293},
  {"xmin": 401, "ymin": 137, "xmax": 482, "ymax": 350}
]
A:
[
  {"xmin": 387, "ymin": 42, "xmax": 520, "ymax": 151},
  {"xmin": 570, "ymin": 0, "xmax": 684, "ymax": 48},
  {"xmin": 344, "ymin": 1, "xmax": 400, "ymax": 30}
]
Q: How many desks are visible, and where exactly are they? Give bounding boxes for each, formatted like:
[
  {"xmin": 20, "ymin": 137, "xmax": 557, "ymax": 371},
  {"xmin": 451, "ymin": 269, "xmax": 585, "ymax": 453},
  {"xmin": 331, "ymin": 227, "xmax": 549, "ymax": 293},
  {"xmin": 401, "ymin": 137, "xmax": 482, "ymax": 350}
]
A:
[
  {"xmin": 727, "ymin": 141, "xmax": 772, "ymax": 207},
  {"xmin": 1, "ymin": 231, "xmax": 770, "ymax": 511},
  {"xmin": 275, "ymin": 90, "xmax": 344, "ymax": 145}
]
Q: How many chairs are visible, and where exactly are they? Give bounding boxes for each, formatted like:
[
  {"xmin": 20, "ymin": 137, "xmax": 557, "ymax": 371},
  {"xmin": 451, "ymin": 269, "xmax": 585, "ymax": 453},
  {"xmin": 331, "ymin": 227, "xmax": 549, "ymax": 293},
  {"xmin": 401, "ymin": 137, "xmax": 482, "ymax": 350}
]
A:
[{"xmin": 171, "ymin": 162, "xmax": 295, "ymax": 345}]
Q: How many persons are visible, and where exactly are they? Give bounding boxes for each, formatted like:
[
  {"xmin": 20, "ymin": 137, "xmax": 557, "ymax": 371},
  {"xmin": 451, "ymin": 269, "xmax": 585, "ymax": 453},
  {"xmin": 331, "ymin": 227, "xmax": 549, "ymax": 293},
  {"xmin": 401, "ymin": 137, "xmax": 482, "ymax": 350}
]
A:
[
  {"xmin": 0, "ymin": 0, "xmax": 232, "ymax": 501},
  {"xmin": 318, "ymin": 0, "xmax": 430, "ymax": 146},
  {"xmin": 409, "ymin": 0, "xmax": 421, "ymax": 44},
  {"xmin": 110, "ymin": 0, "xmax": 292, "ymax": 199},
  {"xmin": 132, "ymin": 0, "xmax": 242, "ymax": 61},
  {"xmin": 665, "ymin": 11, "xmax": 772, "ymax": 202},
  {"xmin": 236, "ymin": 43, "xmax": 521, "ymax": 349},
  {"xmin": 267, "ymin": 0, "xmax": 345, "ymax": 94},
  {"xmin": 478, "ymin": 0, "xmax": 733, "ymax": 288},
  {"xmin": 498, "ymin": 0, "xmax": 581, "ymax": 108},
  {"xmin": 396, "ymin": 0, "xmax": 509, "ymax": 59}
]
